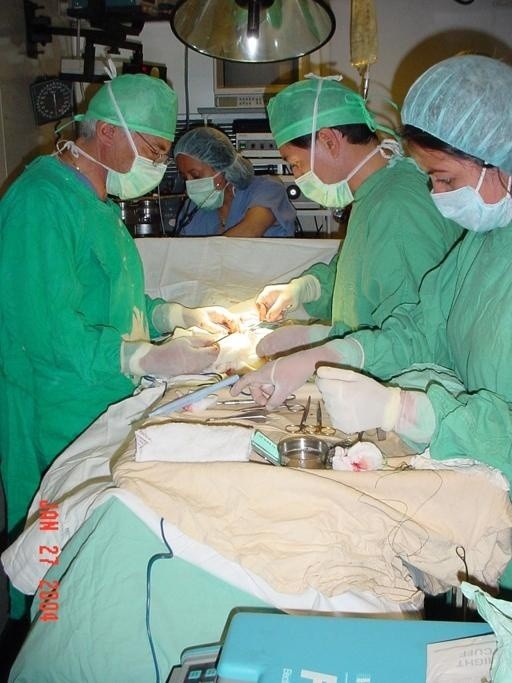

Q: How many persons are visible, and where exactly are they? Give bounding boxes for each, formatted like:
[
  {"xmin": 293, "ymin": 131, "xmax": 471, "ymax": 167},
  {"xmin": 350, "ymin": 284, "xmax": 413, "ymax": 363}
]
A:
[
  {"xmin": 0, "ymin": 57, "xmax": 243, "ymax": 619},
  {"xmin": 257, "ymin": 74, "xmax": 465, "ymax": 355},
  {"xmin": 174, "ymin": 127, "xmax": 298, "ymax": 237},
  {"xmin": 229, "ymin": 54, "xmax": 512, "ymax": 591}
]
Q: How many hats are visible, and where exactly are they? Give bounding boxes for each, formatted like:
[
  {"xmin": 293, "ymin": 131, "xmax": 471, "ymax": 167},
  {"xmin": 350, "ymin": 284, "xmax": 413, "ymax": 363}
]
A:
[
  {"xmin": 265, "ymin": 79, "xmax": 374, "ymax": 149},
  {"xmin": 86, "ymin": 74, "xmax": 177, "ymax": 142},
  {"xmin": 401, "ymin": 55, "xmax": 511, "ymax": 176}
]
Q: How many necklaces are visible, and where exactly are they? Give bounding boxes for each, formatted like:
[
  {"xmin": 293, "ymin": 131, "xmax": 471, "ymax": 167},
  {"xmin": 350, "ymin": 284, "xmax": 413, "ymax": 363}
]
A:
[
  {"xmin": 220, "ymin": 217, "xmax": 227, "ymax": 227},
  {"xmin": 66, "ymin": 152, "xmax": 83, "ymax": 173}
]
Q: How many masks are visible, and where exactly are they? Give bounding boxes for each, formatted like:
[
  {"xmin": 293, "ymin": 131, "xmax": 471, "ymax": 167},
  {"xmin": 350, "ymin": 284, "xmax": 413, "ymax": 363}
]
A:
[
  {"xmin": 293, "ymin": 151, "xmax": 356, "ymax": 209},
  {"xmin": 185, "ymin": 173, "xmax": 227, "ymax": 210},
  {"xmin": 105, "ymin": 145, "xmax": 167, "ymax": 201},
  {"xmin": 428, "ymin": 169, "xmax": 511, "ymax": 233}
]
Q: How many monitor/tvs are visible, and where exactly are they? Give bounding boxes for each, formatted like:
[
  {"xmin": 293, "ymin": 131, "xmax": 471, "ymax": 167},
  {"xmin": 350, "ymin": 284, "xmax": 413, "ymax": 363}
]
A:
[{"xmin": 213, "ymin": 57, "xmax": 295, "ymax": 108}]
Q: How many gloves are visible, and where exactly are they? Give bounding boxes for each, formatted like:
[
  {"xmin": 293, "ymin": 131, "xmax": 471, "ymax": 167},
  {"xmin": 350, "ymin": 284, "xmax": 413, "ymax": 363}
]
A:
[
  {"xmin": 119, "ymin": 338, "xmax": 220, "ymax": 380},
  {"xmin": 313, "ymin": 365, "xmax": 437, "ymax": 445},
  {"xmin": 255, "ymin": 275, "xmax": 322, "ymax": 322},
  {"xmin": 230, "ymin": 335, "xmax": 364, "ymax": 412},
  {"xmin": 256, "ymin": 324, "xmax": 332, "ymax": 362},
  {"xmin": 152, "ymin": 301, "xmax": 240, "ymax": 336}
]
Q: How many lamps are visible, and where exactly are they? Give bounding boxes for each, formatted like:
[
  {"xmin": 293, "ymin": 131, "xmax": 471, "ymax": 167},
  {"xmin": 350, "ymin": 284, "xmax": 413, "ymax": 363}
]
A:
[{"xmin": 170, "ymin": 1, "xmax": 336, "ymax": 64}]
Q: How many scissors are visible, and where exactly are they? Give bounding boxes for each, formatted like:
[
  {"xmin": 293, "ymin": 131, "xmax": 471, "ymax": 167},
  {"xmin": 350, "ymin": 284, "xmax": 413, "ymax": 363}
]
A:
[{"xmin": 208, "ymin": 393, "xmax": 337, "ymax": 436}]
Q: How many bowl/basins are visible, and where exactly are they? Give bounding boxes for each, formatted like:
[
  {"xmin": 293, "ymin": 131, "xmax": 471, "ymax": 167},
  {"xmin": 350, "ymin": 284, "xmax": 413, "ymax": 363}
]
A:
[{"xmin": 278, "ymin": 435, "xmax": 328, "ymax": 469}]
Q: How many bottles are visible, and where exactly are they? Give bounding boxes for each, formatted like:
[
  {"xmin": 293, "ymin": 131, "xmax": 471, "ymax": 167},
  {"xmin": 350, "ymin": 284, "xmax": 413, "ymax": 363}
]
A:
[{"xmin": 118, "ymin": 198, "xmax": 159, "ymax": 237}]
